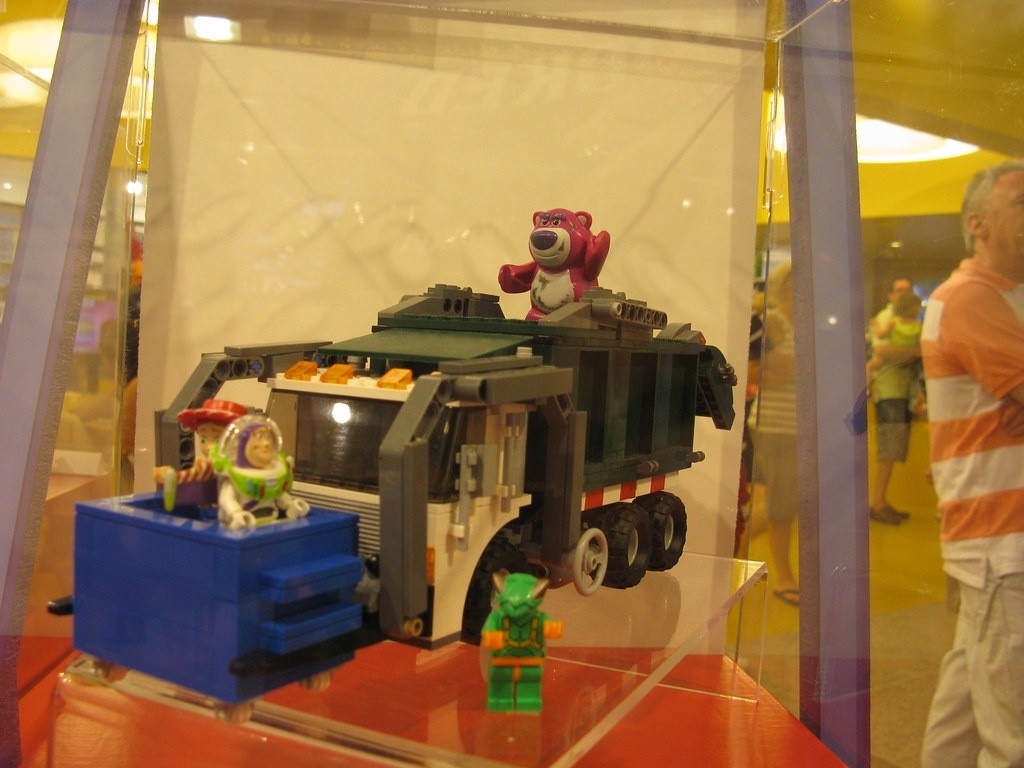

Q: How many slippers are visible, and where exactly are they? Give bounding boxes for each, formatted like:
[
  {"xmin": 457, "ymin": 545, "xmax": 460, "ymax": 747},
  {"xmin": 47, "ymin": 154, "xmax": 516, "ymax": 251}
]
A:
[{"xmin": 772, "ymin": 588, "xmax": 801, "ymax": 606}]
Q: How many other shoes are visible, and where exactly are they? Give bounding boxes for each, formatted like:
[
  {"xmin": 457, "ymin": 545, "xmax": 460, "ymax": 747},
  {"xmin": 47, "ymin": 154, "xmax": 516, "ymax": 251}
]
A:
[
  {"xmin": 870, "ymin": 508, "xmax": 901, "ymax": 524},
  {"xmin": 887, "ymin": 509, "xmax": 910, "ymax": 519}
]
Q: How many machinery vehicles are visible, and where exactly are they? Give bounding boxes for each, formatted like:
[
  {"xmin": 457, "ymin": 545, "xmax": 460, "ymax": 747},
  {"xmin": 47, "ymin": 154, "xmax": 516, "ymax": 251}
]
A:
[{"xmin": 46, "ymin": 283, "xmax": 740, "ymax": 677}]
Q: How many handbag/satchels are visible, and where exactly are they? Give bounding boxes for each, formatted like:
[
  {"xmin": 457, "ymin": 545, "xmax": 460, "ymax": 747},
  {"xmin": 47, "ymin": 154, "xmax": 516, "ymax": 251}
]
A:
[{"xmin": 907, "ymin": 375, "xmax": 927, "ymax": 415}]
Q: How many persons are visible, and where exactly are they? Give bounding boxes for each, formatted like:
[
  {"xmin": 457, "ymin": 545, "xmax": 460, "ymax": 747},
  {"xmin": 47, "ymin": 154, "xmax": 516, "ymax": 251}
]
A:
[
  {"xmin": 748, "ymin": 264, "xmax": 801, "ymax": 605},
  {"xmin": 865, "ymin": 279, "xmax": 922, "ymax": 527},
  {"xmin": 920, "ymin": 163, "xmax": 1024, "ymax": 768}
]
